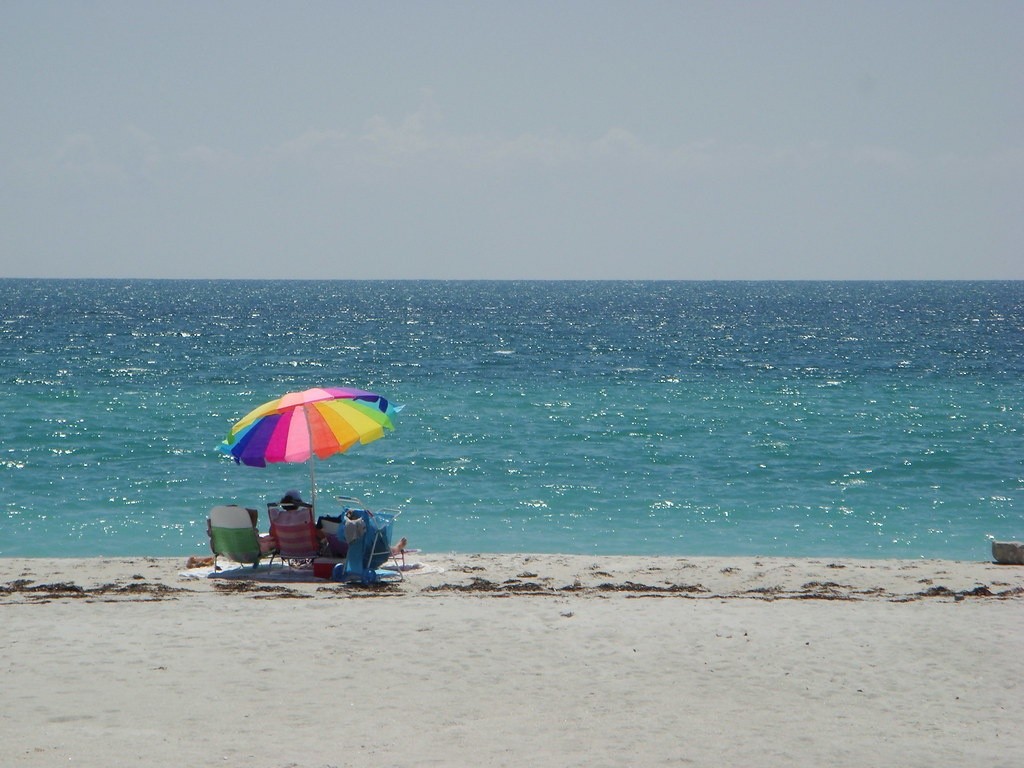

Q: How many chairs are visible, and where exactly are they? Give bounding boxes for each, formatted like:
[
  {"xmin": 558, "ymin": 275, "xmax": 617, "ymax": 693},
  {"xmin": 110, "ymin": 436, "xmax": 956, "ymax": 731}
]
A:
[{"xmin": 210, "ymin": 506, "xmax": 423, "ymax": 579}]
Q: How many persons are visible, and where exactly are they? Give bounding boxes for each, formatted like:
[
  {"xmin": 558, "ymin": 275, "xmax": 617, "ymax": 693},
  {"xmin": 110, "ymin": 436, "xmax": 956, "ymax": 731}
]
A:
[
  {"xmin": 280, "ymin": 490, "xmax": 407, "ymax": 556},
  {"xmin": 229, "ymin": 504, "xmax": 276, "ymax": 552}
]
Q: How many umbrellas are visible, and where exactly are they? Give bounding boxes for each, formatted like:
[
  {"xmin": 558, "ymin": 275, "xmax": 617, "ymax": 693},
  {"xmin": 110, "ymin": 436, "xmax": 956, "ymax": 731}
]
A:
[{"xmin": 215, "ymin": 388, "xmax": 405, "ymax": 523}]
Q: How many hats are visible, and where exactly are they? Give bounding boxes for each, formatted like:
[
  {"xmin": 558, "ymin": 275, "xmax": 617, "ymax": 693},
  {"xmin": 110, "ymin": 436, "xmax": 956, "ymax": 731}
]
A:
[{"xmin": 286, "ymin": 489, "xmax": 303, "ymax": 502}]
